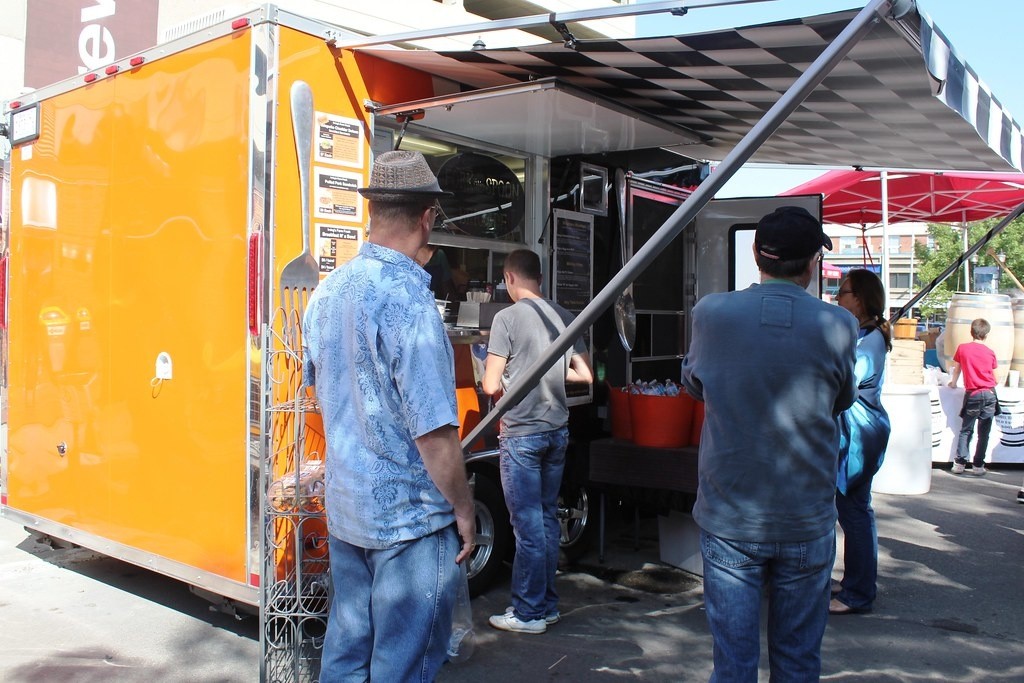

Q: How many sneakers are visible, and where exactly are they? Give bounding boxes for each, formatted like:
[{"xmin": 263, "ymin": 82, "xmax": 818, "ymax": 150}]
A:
[
  {"xmin": 951, "ymin": 459, "xmax": 967, "ymax": 473},
  {"xmin": 972, "ymin": 463, "xmax": 987, "ymax": 475},
  {"xmin": 490, "ymin": 611, "xmax": 546, "ymax": 634},
  {"xmin": 505, "ymin": 603, "xmax": 561, "ymax": 624}
]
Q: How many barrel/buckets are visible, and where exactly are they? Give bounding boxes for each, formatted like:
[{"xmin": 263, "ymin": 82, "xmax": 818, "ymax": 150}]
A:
[
  {"xmin": 871, "ymin": 385, "xmax": 932, "ymax": 495},
  {"xmin": 692, "ymin": 399, "xmax": 705, "ymax": 445},
  {"xmin": 605, "ymin": 379, "xmax": 633, "ymax": 440},
  {"xmin": 924, "ymin": 349, "xmax": 941, "ymax": 370},
  {"xmin": 944, "ymin": 292, "xmax": 1014, "ymax": 386},
  {"xmin": 1011, "ymin": 298, "xmax": 1024, "ymax": 387},
  {"xmin": 894, "ymin": 318, "xmax": 916, "ymax": 338},
  {"xmin": 628, "ymin": 386, "xmax": 694, "ymax": 448}
]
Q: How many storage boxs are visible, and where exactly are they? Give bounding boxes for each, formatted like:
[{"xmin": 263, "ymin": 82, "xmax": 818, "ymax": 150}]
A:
[{"xmin": 657, "ymin": 509, "xmax": 703, "ymax": 577}]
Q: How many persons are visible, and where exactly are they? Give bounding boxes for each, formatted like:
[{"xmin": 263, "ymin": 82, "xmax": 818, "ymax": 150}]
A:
[
  {"xmin": 947, "ymin": 319, "xmax": 999, "ymax": 475},
  {"xmin": 302, "ymin": 150, "xmax": 475, "ymax": 683},
  {"xmin": 483, "ymin": 250, "xmax": 593, "ymax": 633},
  {"xmin": 681, "ymin": 206, "xmax": 859, "ymax": 683},
  {"xmin": 413, "ymin": 213, "xmax": 468, "ymax": 317},
  {"xmin": 827, "ymin": 269, "xmax": 892, "ymax": 613}
]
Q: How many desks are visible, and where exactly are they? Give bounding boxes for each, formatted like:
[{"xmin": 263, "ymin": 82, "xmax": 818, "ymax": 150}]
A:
[{"xmin": 588, "ymin": 437, "xmax": 701, "ymax": 564}]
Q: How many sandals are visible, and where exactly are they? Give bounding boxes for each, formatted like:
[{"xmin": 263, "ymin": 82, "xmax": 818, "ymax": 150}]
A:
[
  {"xmin": 828, "ymin": 597, "xmax": 873, "ymax": 614},
  {"xmin": 830, "ymin": 583, "xmax": 844, "ymax": 594}
]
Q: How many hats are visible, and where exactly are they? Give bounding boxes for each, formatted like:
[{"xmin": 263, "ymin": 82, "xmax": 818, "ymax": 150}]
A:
[
  {"xmin": 357, "ymin": 150, "xmax": 454, "ymax": 203},
  {"xmin": 754, "ymin": 206, "xmax": 832, "ymax": 260}
]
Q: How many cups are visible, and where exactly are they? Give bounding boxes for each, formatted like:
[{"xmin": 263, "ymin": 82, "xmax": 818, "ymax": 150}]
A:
[
  {"xmin": 1009, "ymin": 371, "xmax": 1019, "ymax": 387},
  {"xmin": 435, "ymin": 299, "xmax": 451, "ymax": 318}
]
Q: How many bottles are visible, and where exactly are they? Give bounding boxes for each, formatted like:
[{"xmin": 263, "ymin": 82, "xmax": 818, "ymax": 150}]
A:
[
  {"xmin": 496, "ymin": 279, "xmax": 507, "ymax": 302},
  {"xmin": 481, "ymin": 281, "xmax": 492, "ymax": 302},
  {"xmin": 622, "ymin": 380, "xmax": 643, "ymax": 394},
  {"xmin": 469, "ymin": 281, "xmax": 479, "ymax": 292},
  {"xmin": 643, "ymin": 379, "xmax": 667, "ymax": 396},
  {"xmin": 666, "ymin": 379, "xmax": 678, "ymax": 396}
]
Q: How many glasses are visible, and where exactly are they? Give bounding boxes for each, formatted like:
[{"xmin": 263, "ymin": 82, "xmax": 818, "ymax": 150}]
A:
[
  {"xmin": 817, "ymin": 250, "xmax": 824, "ymax": 262},
  {"xmin": 839, "ymin": 289, "xmax": 853, "ymax": 297}
]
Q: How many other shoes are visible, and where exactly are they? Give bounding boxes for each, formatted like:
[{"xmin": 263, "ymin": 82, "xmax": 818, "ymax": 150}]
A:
[{"xmin": 1016, "ymin": 490, "xmax": 1024, "ymax": 502}]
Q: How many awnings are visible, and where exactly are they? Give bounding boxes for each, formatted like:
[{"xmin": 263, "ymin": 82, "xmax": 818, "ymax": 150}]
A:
[
  {"xmin": 333, "ymin": 0, "xmax": 1024, "ymax": 449},
  {"xmin": 758, "ymin": 166, "xmax": 1024, "ymax": 320}
]
